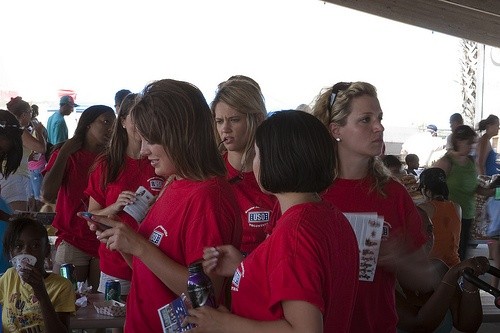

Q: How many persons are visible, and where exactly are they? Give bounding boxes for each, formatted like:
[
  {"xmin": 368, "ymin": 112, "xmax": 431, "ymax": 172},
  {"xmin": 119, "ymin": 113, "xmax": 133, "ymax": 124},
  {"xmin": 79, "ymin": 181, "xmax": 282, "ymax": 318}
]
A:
[
  {"xmin": 180, "ymin": 109, "xmax": 360, "ymax": 333},
  {"xmin": 84, "ymin": 93, "xmax": 168, "ymax": 303},
  {"xmin": 0, "ymin": 90, "xmax": 132, "ymax": 290},
  {"xmin": 295, "ymin": 103, "xmax": 500, "ymax": 333},
  {"xmin": 313, "ymin": 82, "xmax": 435, "ymax": 333},
  {"xmin": 210, "ymin": 76, "xmax": 282, "ymax": 257},
  {"xmin": 91, "ymin": 80, "xmax": 243, "ymax": 333},
  {"xmin": 0, "ymin": 217, "xmax": 75, "ymax": 333}
]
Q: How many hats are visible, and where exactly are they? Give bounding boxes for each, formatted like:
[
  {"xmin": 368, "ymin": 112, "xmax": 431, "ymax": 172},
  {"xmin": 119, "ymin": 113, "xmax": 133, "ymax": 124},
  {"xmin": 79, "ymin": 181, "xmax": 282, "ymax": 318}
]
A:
[{"xmin": 60, "ymin": 95, "xmax": 79, "ymax": 107}]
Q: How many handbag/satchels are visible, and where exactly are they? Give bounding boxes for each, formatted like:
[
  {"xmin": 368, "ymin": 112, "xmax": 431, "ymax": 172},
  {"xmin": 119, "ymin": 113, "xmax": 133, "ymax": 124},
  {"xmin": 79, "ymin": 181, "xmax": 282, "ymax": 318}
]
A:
[{"xmin": 27, "ymin": 150, "xmax": 45, "ymax": 200}]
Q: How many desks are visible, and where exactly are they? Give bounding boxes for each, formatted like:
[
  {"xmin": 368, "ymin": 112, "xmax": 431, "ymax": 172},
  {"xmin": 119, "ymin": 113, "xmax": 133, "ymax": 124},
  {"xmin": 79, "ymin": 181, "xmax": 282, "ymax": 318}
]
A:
[{"xmin": 69, "ymin": 290, "xmax": 128, "ymax": 333}]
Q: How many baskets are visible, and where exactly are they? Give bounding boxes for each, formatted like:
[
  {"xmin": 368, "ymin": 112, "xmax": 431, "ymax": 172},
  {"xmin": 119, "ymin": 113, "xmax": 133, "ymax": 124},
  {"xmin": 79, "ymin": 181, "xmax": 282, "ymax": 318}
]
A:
[{"xmin": 11, "ymin": 200, "xmax": 56, "ymax": 225}]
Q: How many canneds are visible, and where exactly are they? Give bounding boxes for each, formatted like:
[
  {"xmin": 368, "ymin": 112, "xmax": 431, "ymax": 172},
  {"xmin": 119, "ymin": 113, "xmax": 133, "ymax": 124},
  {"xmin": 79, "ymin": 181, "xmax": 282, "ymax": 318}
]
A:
[
  {"xmin": 59, "ymin": 263, "xmax": 78, "ymax": 291},
  {"xmin": 105, "ymin": 280, "xmax": 122, "ymax": 302}
]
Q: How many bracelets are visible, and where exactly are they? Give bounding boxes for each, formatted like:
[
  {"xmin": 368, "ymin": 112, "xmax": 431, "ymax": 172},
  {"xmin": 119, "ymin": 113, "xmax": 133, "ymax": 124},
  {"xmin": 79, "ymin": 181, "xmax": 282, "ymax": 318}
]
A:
[
  {"xmin": 450, "ymin": 265, "xmax": 463, "ymax": 277},
  {"xmin": 441, "ymin": 281, "xmax": 458, "ymax": 288},
  {"xmin": 459, "ymin": 280, "xmax": 477, "ymax": 294}
]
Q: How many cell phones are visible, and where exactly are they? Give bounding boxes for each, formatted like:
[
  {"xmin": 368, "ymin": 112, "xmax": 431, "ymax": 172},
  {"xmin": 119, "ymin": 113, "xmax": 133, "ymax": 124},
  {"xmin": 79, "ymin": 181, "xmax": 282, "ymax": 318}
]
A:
[{"xmin": 76, "ymin": 212, "xmax": 112, "ymax": 238}]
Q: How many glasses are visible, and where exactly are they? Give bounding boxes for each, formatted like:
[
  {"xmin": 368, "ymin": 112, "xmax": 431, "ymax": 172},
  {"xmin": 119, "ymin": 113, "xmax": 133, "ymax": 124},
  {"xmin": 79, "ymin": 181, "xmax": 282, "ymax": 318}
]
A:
[
  {"xmin": 217, "ymin": 138, "xmax": 253, "ymax": 185},
  {"xmin": 327, "ymin": 82, "xmax": 354, "ymax": 123}
]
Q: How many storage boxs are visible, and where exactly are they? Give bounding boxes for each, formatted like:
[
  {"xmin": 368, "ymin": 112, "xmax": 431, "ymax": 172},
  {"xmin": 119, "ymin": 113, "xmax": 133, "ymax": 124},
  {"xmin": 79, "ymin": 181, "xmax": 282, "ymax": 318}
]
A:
[{"xmin": 93, "ymin": 300, "xmax": 128, "ymax": 317}]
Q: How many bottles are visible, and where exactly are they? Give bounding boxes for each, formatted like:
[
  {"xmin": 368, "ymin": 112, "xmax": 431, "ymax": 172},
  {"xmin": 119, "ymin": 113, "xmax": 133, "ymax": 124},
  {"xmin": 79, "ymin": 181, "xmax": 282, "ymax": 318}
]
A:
[{"xmin": 188, "ymin": 263, "xmax": 216, "ymax": 310}]
